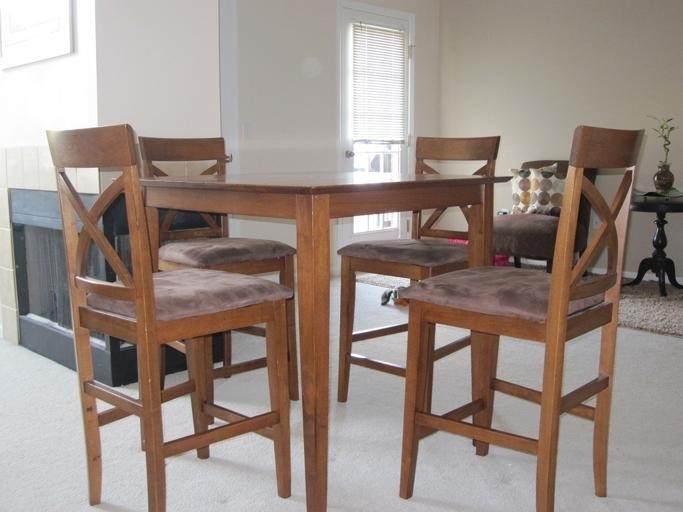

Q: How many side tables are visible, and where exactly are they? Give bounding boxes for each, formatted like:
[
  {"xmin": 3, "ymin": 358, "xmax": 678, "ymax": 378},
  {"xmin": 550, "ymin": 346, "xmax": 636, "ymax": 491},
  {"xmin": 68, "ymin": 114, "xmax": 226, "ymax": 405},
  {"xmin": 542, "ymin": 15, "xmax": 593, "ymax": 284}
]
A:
[{"xmin": 621, "ymin": 191, "xmax": 682, "ymax": 299}]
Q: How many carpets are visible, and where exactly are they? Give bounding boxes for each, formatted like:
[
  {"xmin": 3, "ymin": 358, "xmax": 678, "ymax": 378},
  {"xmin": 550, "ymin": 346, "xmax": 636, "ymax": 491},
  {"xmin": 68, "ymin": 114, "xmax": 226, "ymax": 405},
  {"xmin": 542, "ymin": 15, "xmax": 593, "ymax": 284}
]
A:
[{"xmin": 355, "ymin": 261, "xmax": 682, "ymax": 341}]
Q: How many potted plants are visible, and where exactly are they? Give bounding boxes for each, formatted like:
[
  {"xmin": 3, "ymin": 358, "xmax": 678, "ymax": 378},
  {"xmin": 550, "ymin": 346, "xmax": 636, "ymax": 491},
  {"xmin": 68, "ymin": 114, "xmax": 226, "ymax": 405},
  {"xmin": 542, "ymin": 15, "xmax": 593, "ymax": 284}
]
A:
[{"xmin": 649, "ymin": 111, "xmax": 680, "ymax": 190}]
